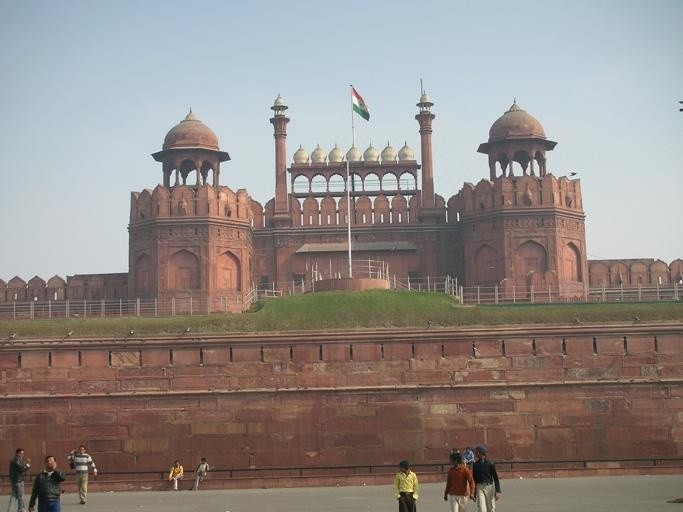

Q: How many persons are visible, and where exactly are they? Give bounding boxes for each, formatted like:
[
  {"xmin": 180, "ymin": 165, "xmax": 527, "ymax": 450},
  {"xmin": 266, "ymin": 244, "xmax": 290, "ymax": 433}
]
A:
[
  {"xmin": 442, "ymin": 452, "xmax": 475, "ymax": 512},
  {"xmin": 462, "ymin": 447, "xmax": 474, "ymax": 475},
  {"xmin": 390, "ymin": 458, "xmax": 420, "ymax": 512},
  {"xmin": 472, "ymin": 445, "xmax": 502, "ymax": 512},
  {"xmin": 66, "ymin": 445, "xmax": 99, "ymax": 504},
  {"xmin": 6, "ymin": 447, "xmax": 32, "ymax": 512},
  {"xmin": 189, "ymin": 456, "xmax": 210, "ymax": 490},
  {"xmin": 167, "ymin": 459, "xmax": 184, "ymax": 490},
  {"xmin": 27, "ymin": 455, "xmax": 66, "ymax": 512}
]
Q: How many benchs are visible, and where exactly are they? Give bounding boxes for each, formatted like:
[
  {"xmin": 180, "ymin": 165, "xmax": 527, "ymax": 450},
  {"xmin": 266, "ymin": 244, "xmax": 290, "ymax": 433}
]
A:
[{"xmin": 351, "ymin": 87, "xmax": 369, "ymax": 121}]
